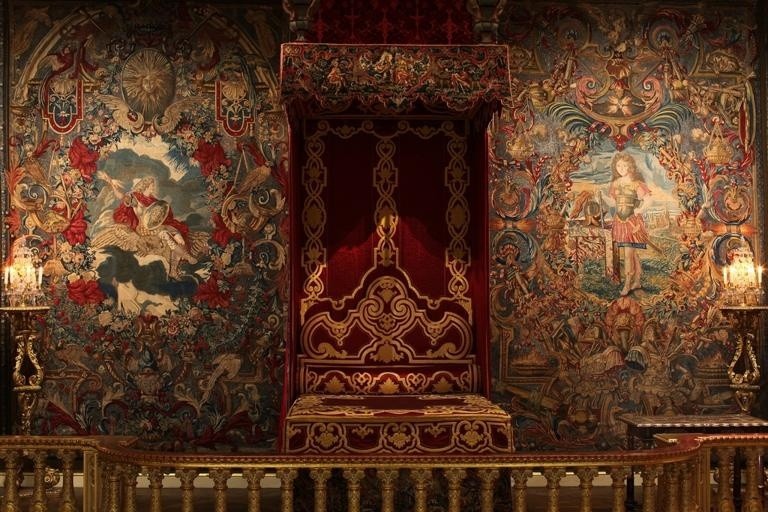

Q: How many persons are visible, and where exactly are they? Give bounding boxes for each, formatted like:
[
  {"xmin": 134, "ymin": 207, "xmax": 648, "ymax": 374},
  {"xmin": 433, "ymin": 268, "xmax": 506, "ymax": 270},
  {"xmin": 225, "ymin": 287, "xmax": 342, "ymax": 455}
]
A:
[
  {"xmin": 596, "ymin": 150, "xmax": 652, "ymax": 297},
  {"xmin": 102, "ymin": 172, "xmax": 199, "ymax": 284}
]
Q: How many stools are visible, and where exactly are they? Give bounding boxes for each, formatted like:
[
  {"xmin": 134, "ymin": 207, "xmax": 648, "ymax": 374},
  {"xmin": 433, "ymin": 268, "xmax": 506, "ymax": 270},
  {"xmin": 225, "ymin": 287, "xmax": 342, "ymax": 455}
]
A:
[{"xmin": 616, "ymin": 411, "xmax": 767, "ymax": 511}]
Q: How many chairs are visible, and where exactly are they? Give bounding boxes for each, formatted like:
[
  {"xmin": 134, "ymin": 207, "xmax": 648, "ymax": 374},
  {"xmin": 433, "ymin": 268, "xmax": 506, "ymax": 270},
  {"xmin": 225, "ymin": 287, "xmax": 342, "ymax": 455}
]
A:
[{"xmin": 277, "ymin": 39, "xmax": 517, "ymax": 456}]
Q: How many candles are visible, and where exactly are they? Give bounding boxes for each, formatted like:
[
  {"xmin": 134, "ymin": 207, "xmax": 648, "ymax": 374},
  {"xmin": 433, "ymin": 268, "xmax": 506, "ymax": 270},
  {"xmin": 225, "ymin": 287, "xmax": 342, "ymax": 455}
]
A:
[
  {"xmin": 0, "ymin": 265, "xmax": 46, "ymax": 285},
  {"xmin": 720, "ymin": 263, "xmax": 764, "ymax": 286}
]
